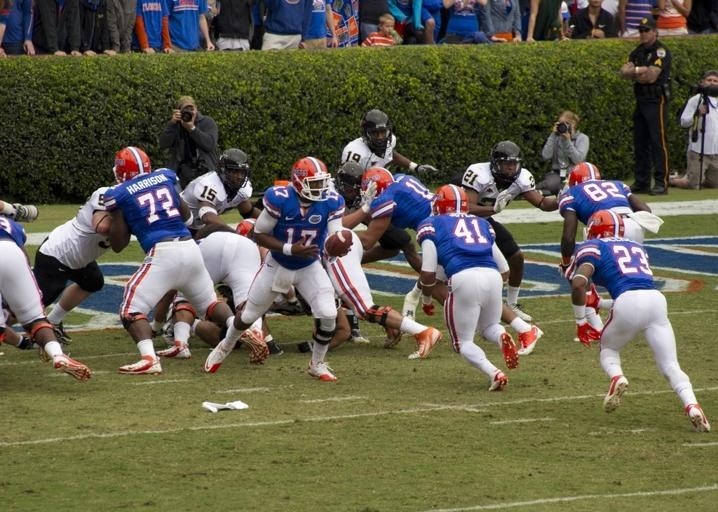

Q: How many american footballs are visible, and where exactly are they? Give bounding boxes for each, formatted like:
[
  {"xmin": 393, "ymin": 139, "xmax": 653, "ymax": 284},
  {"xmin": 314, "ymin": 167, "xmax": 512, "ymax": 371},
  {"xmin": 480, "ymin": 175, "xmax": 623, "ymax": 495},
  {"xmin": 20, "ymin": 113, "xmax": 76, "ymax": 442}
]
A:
[{"xmin": 325, "ymin": 230, "xmax": 352, "ymax": 257}]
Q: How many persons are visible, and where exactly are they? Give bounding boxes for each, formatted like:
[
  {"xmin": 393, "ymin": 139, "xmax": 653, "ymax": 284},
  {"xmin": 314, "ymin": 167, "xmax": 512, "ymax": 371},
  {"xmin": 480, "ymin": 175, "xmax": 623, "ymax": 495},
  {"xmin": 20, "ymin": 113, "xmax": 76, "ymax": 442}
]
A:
[
  {"xmin": 351, "ymin": 166, "xmax": 544, "ymax": 356},
  {"xmin": 161, "ymin": 96, "xmax": 218, "ymax": 190},
  {"xmin": 460, "ymin": 141, "xmax": 559, "ymax": 322},
  {"xmin": 1, "ymin": 201, "xmax": 91, "ymax": 383},
  {"xmin": 669, "ymin": 70, "xmax": 717, "ymax": 190},
  {"xmin": 363, "ymin": 0, "xmax": 718, "ymax": 45},
  {"xmin": 414, "ymin": 185, "xmax": 519, "ymax": 391},
  {"xmin": 340, "ymin": 110, "xmax": 422, "ymax": 274},
  {"xmin": 619, "ymin": 16, "xmax": 673, "ymax": 196},
  {"xmin": 201, "ymin": 157, "xmax": 353, "ymax": 382},
  {"xmin": 35, "ymin": 151, "xmax": 442, "ymax": 360},
  {"xmin": 542, "ymin": 111, "xmax": 589, "ymax": 184},
  {"xmin": 557, "ymin": 162, "xmax": 652, "ymax": 340},
  {"xmin": 106, "ymin": 146, "xmax": 269, "ymax": 376},
  {"xmin": 573, "ymin": 208, "xmax": 711, "ymax": 432},
  {"xmin": 2, "ymin": 1, "xmax": 337, "ymax": 56}
]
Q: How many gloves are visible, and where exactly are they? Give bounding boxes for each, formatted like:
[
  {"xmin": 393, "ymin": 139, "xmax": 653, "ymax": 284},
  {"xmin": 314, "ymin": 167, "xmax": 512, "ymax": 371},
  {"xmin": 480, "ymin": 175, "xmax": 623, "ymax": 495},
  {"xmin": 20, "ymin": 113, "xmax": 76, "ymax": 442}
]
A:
[
  {"xmin": 582, "ymin": 283, "xmax": 601, "ymax": 313},
  {"xmin": 573, "ymin": 322, "xmax": 605, "ymax": 350}
]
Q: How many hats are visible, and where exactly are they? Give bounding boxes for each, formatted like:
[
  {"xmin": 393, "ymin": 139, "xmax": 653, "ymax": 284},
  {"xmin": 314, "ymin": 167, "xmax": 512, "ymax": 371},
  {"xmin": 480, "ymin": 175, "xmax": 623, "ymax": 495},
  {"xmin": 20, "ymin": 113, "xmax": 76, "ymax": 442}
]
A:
[
  {"xmin": 176, "ymin": 96, "xmax": 195, "ymax": 110},
  {"xmin": 636, "ymin": 17, "xmax": 656, "ymax": 29}
]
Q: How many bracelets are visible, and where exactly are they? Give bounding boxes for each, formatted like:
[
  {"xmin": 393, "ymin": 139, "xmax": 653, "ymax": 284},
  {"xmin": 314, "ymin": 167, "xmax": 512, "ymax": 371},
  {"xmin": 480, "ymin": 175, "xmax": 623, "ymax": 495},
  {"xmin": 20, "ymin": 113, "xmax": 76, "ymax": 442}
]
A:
[{"xmin": 634, "ymin": 66, "xmax": 639, "ymax": 78}]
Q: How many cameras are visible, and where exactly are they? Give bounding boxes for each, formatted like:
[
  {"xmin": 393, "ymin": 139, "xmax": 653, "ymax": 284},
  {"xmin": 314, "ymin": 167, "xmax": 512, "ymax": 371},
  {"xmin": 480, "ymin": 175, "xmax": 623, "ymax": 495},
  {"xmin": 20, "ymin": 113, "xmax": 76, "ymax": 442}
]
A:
[
  {"xmin": 556, "ymin": 121, "xmax": 572, "ymax": 134},
  {"xmin": 690, "ymin": 82, "xmax": 718, "ymax": 98},
  {"xmin": 177, "ymin": 110, "xmax": 192, "ymax": 123}
]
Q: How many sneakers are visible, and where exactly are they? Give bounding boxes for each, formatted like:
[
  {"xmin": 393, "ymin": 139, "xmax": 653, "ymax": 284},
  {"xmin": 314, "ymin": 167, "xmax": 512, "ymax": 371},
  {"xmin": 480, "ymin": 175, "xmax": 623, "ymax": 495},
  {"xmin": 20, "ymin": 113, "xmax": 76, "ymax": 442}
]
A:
[
  {"xmin": 272, "ymin": 297, "xmax": 307, "ymax": 316},
  {"xmin": 238, "ymin": 324, "xmax": 284, "ymax": 364},
  {"xmin": 383, "ymin": 294, "xmax": 419, "ymax": 349},
  {"xmin": 149, "ymin": 302, "xmax": 174, "ymax": 337},
  {"xmin": 51, "ymin": 353, "xmax": 92, "ymax": 381},
  {"xmin": 16, "ymin": 334, "xmax": 40, "ymax": 350},
  {"xmin": 408, "ymin": 326, "xmax": 443, "ymax": 360},
  {"xmin": 630, "ymin": 170, "xmax": 679, "ymax": 196},
  {"xmin": 155, "ymin": 341, "xmax": 192, "ymax": 360},
  {"xmin": 684, "ymin": 403, "xmax": 712, "ymax": 432},
  {"xmin": 347, "ymin": 324, "xmax": 370, "ymax": 346},
  {"xmin": 294, "ymin": 340, "xmax": 313, "ymax": 353},
  {"xmin": 517, "ymin": 324, "xmax": 544, "ymax": 355},
  {"xmin": 46, "ymin": 316, "xmax": 72, "ymax": 345},
  {"xmin": 499, "ymin": 333, "xmax": 519, "ymax": 370},
  {"xmin": 117, "ymin": 355, "xmax": 163, "ymax": 376},
  {"xmin": 488, "ymin": 368, "xmax": 508, "ymax": 392},
  {"xmin": 304, "ymin": 358, "xmax": 338, "ymax": 383},
  {"xmin": 602, "ymin": 375, "xmax": 629, "ymax": 413},
  {"xmin": 204, "ymin": 338, "xmax": 236, "ymax": 373}
]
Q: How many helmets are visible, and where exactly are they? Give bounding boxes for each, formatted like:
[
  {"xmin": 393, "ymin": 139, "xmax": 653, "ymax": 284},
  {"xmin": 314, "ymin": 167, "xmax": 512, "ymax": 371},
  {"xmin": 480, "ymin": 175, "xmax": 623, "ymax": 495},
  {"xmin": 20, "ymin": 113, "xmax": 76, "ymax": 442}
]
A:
[
  {"xmin": 334, "ymin": 160, "xmax": 395, "ymax": 206},
  {"xmin": 112, "ymin": 146, "xmax": 151, "ymax": 184},
  {"xmin": 567, "ymin": 161, "xmax": 601, "ymax": 189},
  {"xmin": 431, "ymin": 183, "xmax": 470, "ymax": 216},
  {"xmin": 235, "ymin": 217, "xmax": 258, "ymax": 242},
  {"xmin": 290, "ymin": 157, "xmax": 332, "ymax": 202},
  {"xmin": 214, "ymin": 148, "xmax": 251, "ymax": 189},
  {"xmin": 489, "ymin": 139, "xmax": 523, "ymax": 188},
  {"xmin": 360, "ymin": 108, "xmax": 394, "ymax": 150},
  {"xmin": 583, "ymin": 209, "xmax": 625, "ymax": 240}
]
